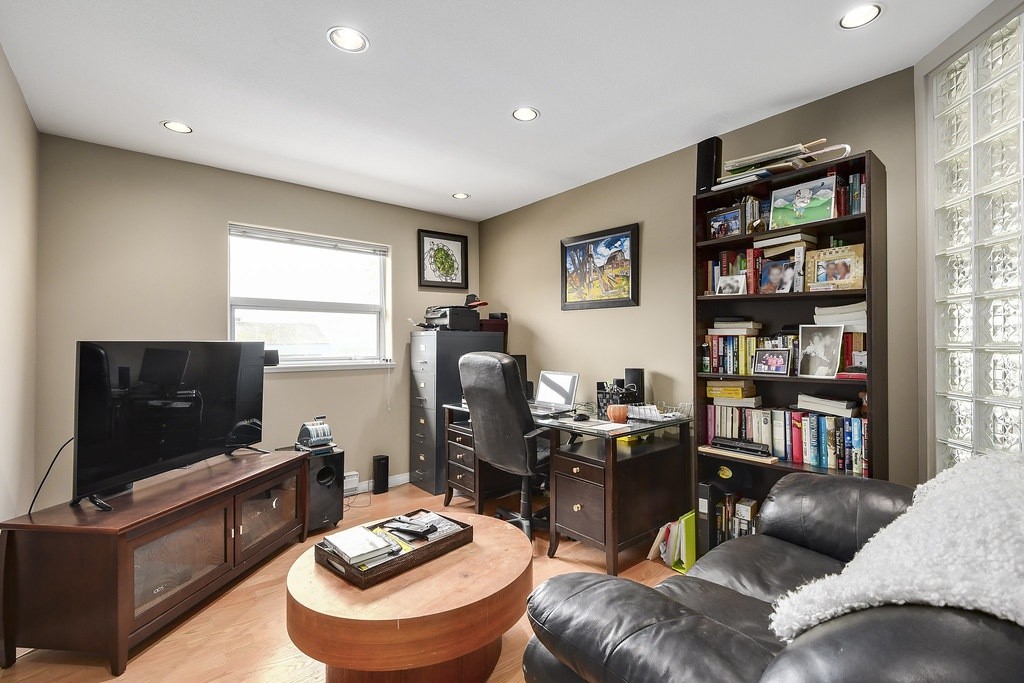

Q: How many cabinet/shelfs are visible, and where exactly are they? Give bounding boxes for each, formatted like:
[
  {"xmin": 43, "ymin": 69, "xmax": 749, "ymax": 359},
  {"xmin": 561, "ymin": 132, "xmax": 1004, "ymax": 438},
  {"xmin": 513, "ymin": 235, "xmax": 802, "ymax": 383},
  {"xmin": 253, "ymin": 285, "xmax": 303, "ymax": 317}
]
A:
[
  {"xmin": 690, "ymin": 147, "xmax": 890, "ymax": 564},
  {"xmin": 440, "ymin": 395, "xmax": 695, "ymax": 579},
  {"xmin": 408, "ymin": 330, "xmax": 505, "ymax": 500},
  {"xmin": 0, "ymin": 447, "xmax": 310, "ymax": 676}
]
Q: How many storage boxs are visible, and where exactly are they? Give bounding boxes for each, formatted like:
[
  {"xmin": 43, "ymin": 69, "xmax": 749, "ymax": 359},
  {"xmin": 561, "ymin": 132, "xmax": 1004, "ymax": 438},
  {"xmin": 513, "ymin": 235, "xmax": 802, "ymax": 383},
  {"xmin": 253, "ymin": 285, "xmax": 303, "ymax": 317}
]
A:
[
  {"xmin": 706, "ymin": 386, "xmax": 755, "ymax": 399},
  {"xmin": 735, "ymin": 496, "xmax": 757, "ymax": 520},
  {"xmin": 480, "ymin": 319, "xmax": 509, "ymax": 332},
  {"xmin": 696, "ymin": 481, "xmax": 712, "ymax": 556}
]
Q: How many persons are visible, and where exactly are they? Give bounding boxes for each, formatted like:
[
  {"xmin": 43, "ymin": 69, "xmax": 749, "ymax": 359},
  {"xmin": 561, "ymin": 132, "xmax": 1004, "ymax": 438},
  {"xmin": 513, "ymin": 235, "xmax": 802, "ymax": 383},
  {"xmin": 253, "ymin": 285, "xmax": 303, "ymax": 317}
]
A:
[
  {"xmin": 770, "ymin": 354, "xmax": 778, "ymax": 371},
  {"xmin": 835, "ymin": 261, "xmax": 851, "ymax": 280},
  {"xmin": 728, "ymin": 277, "xmax": 734, "ymax": 288},
  {"xmin": 818, "ymin": 261, "xmax": 838, "ymax": 282},
  {"xmin": 718, "ymin": 280, "xmax": 727, "ymax": 294},
  {"xmin": 723, "ymin": 284, "xmax": 733, "ymax": 294},
  {"xmin": 775, "ymin": 354, "xmax": 784, "ymax": 371},
  {"xmin": 760, "ymin": 264, "xmax": 782, "ymax": 293},
  {"xmin": 762, "ymin": 353, "xmax": 769, "ymax": 371},
  {"xmin": 732, "ymin": 280, "xmax": 740, "ymax": 293},
  {"xmin": 777, "ymin": 262, "xmax": 795, "ymax": 293},
  {"xmin": 767, "ymin": 355, "xmax": 773, "ymax": 370}
]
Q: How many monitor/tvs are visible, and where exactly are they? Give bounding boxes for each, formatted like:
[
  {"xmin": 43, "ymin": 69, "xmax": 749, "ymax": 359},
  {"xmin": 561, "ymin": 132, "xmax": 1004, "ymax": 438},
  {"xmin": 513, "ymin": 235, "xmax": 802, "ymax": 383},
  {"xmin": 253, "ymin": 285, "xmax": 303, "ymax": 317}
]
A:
[{"xmin": 69, "ymin": 341, "xmax": 271, "ymax": 511}]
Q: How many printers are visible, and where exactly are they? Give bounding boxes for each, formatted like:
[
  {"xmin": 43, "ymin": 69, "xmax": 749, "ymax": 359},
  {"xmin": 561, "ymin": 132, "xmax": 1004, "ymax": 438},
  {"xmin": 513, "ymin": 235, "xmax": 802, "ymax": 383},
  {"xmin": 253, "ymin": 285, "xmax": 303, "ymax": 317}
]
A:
[{"xmin": 408, "ymin": 304, "xmax": 480, "ymax": 332}]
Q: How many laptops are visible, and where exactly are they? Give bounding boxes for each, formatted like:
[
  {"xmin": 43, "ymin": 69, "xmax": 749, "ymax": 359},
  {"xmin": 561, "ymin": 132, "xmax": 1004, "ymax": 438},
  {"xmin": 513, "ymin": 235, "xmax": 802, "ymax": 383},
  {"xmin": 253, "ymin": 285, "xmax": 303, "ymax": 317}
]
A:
[{"xmin": 528, "ymin": 371, "xmax": 580, "ymax": 416}]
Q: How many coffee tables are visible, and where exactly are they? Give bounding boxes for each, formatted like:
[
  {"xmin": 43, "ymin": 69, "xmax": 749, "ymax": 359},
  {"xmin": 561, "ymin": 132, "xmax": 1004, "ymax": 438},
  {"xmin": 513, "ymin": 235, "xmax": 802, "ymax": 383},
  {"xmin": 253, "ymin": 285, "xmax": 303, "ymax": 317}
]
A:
[{"xmin": 284, "ymin": 511, "xmax": 538, "ymax": 683}]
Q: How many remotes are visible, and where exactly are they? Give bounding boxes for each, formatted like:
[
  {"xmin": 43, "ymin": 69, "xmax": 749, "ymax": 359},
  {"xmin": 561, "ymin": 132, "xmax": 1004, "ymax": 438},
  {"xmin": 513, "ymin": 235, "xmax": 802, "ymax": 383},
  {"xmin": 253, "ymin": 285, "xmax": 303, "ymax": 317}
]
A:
[
  {"xmin": 394, "ymin": 515, "xmax": 438, "ymax": 533},
  {"xmin": 375, "ymin": 531, "xmax": 402, "ymax": 555},
  {"xmin": 384, "ymin": 522, "xmax": 430, "ymax": 537}
]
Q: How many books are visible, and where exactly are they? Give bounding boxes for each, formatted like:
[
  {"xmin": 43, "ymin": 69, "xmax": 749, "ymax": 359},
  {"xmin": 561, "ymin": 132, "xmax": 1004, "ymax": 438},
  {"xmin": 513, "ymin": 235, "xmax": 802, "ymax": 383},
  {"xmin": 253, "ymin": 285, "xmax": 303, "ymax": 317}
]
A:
[
  {"xmin": 706, "ymin": 186, "xmax": 771, "ymax": 239},
  {"xmin": 813, "ymin": 301, "xmax": 868, "ymax": 379},
  {"xmin": 323, "ymin": 525, "xmax": 413, "ymax": 572},
  {"xmin": 827, "ymin": 171, "xmax": 866, "ymax": 218},
  {"xmin": 710, "ymin": 143, "xmax": 818, "ymax": 191},
  {"xmin": 647, "ymin": 469, "xmax": 759, "ymax": 575},
  {"xmin": 415, "ymin": 511, "xmax": 462, "ymax": 541},
  {"xmin": 701, "ymin": 321, "xmax": 799, "ymax": 374},
  {"xmin": 707, "ymin": 380, "xmax": 868, "ymax": 477},
  {"xmin": 707, "ymin": 233, "xmax": 851, "ymax": 295}
]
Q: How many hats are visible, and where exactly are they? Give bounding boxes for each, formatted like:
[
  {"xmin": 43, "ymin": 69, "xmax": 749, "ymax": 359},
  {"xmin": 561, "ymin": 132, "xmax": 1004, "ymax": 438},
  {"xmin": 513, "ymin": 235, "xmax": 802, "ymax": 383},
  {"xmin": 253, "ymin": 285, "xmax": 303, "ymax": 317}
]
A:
[{"xmin": 465, "ymin": 294, "xmax": 488, "ymax": 308}]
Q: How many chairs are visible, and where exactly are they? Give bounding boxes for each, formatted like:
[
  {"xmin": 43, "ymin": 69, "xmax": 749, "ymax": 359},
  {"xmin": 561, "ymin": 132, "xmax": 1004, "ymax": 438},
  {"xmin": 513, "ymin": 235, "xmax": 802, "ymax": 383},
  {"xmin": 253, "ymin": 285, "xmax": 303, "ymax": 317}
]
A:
[{"xmin": 457, "ymin": 349, "xmax": 575, "ymax": 549}]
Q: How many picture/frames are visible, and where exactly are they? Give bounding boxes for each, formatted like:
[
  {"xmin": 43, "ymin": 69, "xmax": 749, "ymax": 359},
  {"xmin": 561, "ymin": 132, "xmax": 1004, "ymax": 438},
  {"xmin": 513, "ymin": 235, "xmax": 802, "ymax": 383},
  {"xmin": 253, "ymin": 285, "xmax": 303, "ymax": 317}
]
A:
[
  {"xmin": 418, "ymin": 228, "xmax": 470, "ymax": 290},
  {"xmin": 706, "ymin": 205, "xmax": 748, "ymax": 239},
  {"xmin": 751, "ymin": 346, "xmax": 793, "ymax": 378},
  {"xmin": 804, "ymin": 243, "xmax": 864, "ymax": 291},
  {"xmin": 560, "ymin": 220, "xmax": 642, "ymax": 310}
]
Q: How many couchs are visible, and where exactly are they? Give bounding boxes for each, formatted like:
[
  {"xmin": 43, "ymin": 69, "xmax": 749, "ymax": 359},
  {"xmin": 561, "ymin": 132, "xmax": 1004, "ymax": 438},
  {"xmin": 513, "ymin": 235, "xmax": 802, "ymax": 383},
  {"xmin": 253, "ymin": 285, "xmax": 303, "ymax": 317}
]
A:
[{"xmin": 519, "ymin": 462, "xmax": 1024, "ymax": 683}]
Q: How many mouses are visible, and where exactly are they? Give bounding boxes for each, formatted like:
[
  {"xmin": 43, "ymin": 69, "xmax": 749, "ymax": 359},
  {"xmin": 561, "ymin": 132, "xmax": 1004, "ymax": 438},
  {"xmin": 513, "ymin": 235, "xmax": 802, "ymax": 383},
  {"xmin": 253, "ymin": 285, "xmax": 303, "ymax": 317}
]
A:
[{"xmin": 573, "ymin": 414, "xmax": 590, "ymax": 421}]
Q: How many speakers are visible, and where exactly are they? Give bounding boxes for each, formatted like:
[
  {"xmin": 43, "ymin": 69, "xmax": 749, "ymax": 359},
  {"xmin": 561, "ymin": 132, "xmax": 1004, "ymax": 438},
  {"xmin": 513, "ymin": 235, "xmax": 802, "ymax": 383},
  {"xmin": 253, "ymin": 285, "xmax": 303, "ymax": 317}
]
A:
[
  {"xmin": 275, "ymin": 446, "xmax": 342, "ymax": 532},
  {"xmin": 696, "ymin": 136, "xmax": 723, "ymax": 195},
  {"xmin": 624, "ymin": 368, "xmax": 645, "ymax": 402},
  {"xmin": 372, "ymin": 455, "xmax": 389, "ymax": 494}
]
